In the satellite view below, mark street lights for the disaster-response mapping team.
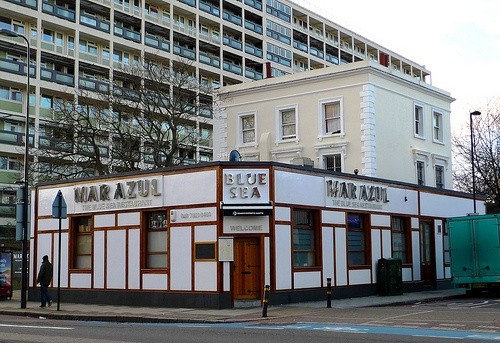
[0,29,32,308]
[470,111,482,214]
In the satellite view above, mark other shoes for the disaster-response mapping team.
[48,300,52,307]
[39,305,45,308]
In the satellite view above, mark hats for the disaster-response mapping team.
[42,255,47,261]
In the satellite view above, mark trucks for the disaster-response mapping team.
[446,213,500,298]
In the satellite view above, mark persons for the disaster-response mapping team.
[36,255,54,308]
